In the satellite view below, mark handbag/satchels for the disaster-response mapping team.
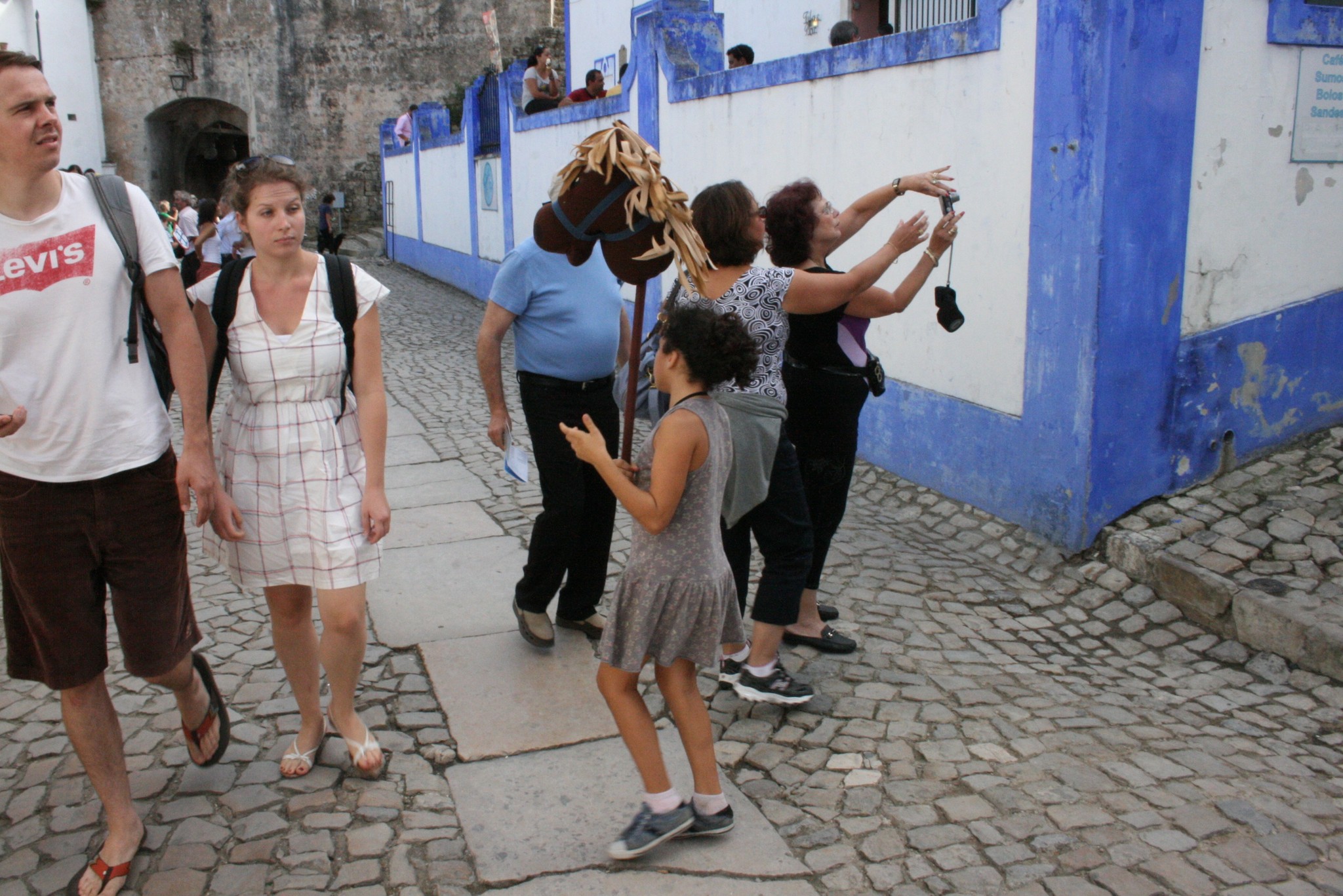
[173,245,184,258]
[863,352,888,397]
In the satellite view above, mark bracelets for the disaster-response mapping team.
[886,242,898,264]
[925,247,939,268]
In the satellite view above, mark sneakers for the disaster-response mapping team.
[608,802,696,861]
[719,640,784,691]
[673,799,737,838]
[513,595,556,647]
[555,610,608,640]
[733,665,814,707]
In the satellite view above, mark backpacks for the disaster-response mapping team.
[86,171,176,414]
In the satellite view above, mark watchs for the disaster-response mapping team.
[892,178,905,196]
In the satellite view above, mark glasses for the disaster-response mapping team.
[856,35,861,40]
[750,207,769,218]
[234,153,296,173]
[819,200,833,216]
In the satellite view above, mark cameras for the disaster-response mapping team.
[940,190,959,218]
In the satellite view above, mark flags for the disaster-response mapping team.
[482,10,503,73]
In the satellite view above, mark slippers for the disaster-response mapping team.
[179,652,231,768]
[67,824,148,896]
[326,701,386,778]
[279,714,328,780]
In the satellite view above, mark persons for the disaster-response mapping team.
[185,154,389,779]
[0,51,232,896]
[521,47,562,115]
[726,44,754,68]
[768,165,966,650]
[561,297,758,860]
[830,21,861,47]
[63,164,337,285]
[669,179,929,705]
[558,70,607,108]
[605,63,629,96]
[394,104,418,147]
[479,233,639,647]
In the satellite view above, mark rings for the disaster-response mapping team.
[948,229,955,236]
[918,228,924,235]
[932,172,938,184]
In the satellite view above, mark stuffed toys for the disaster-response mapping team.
[533,121,719,296]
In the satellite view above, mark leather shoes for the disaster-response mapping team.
[781,624,856,654]
[815,600,838,621]
